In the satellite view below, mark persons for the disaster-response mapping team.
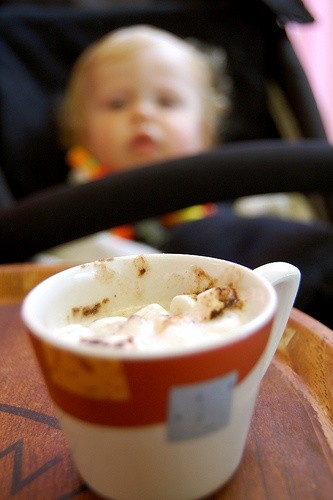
[39,19,316,265]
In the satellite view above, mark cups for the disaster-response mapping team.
[20,252,301,500]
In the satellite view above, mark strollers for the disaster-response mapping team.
[0,0,333,331]
